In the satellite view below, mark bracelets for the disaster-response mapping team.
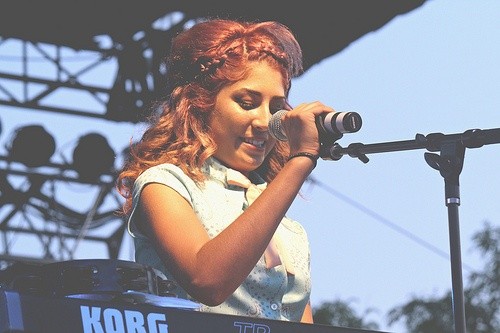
[287,149,318,174]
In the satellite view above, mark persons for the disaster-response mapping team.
[109,16,335,324]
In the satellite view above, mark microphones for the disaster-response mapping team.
[268,109,363,142]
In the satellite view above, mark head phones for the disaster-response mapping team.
[1,261,178,305]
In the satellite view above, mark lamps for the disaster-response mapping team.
[5,123,57,168]
[71,133,116,182]
